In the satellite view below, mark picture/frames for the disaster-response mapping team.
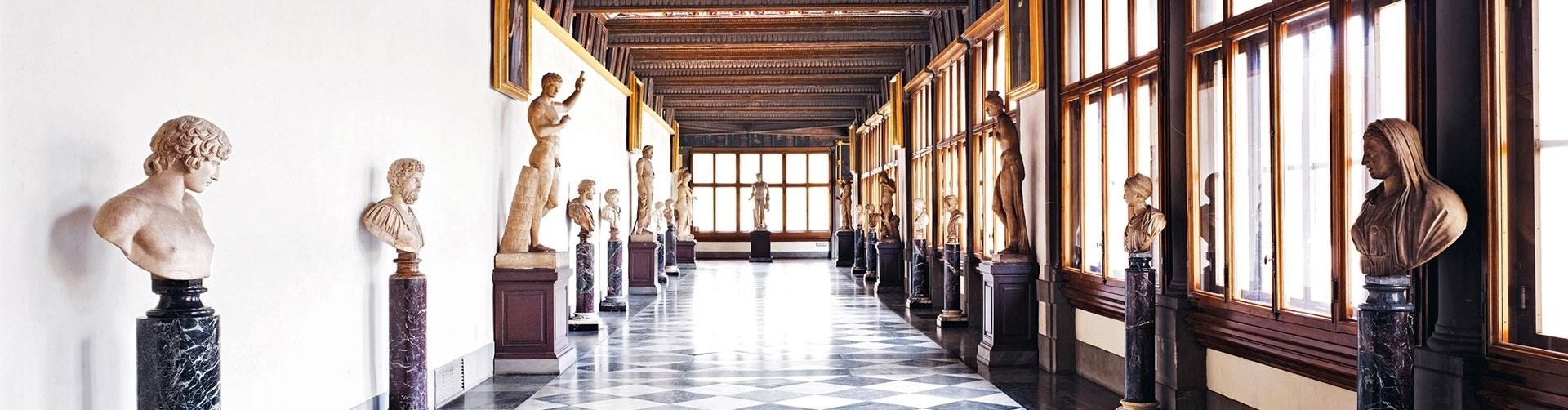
[491,0,533,103]
[1002,0,1044,102]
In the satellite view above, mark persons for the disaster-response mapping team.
[748,173,772,229]
[910,197,930,239]
[1350,118,1470,276]
[358,159,430,254]
[525,70,698,258]
[985,90,1032,255]
[1123,173,1168,254]
[1198,172,1215,252]
[92,115,235,280]
[837,171,897,239]
[944,194,965,243]
